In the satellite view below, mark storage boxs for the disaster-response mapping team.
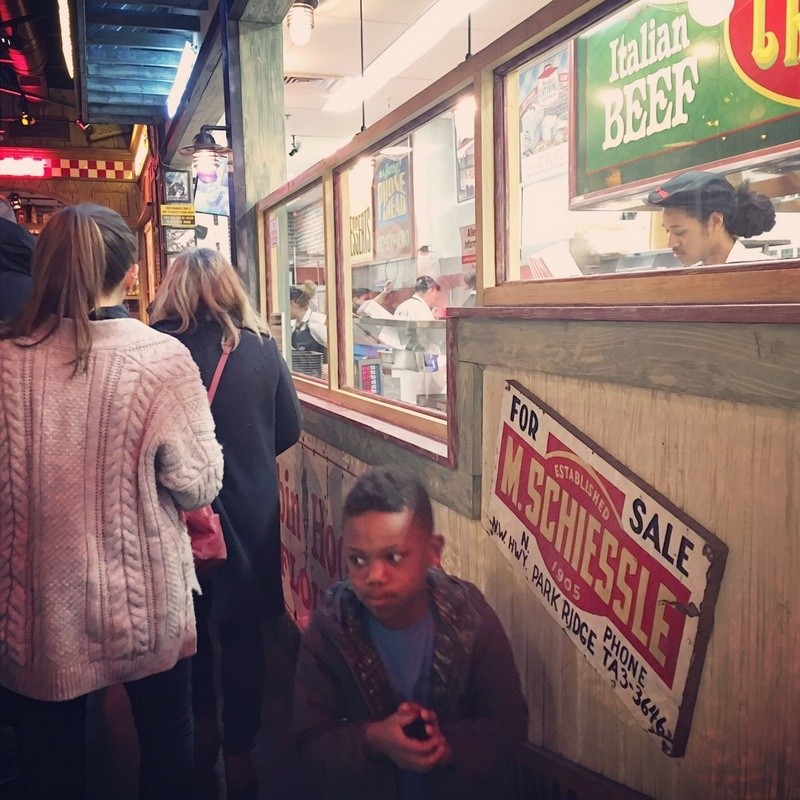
[357,299,411,349]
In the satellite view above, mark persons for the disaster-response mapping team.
[285,267,480,367]
[138,243,307,789]
[640,169,780,272]
[286,464,529,800]
[0,202,224,800]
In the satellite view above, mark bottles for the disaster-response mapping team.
[31,203,37,224]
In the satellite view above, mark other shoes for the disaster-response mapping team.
[192,718,218,776]
[220,749,261,800]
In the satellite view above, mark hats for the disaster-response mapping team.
[647,171,736,218]
[415,276,441,291]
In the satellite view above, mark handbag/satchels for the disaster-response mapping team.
[184,503,227,568]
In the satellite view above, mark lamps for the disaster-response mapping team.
[74,118,91,130]
[176,123,233,185]
[10,192,22,209]
[284,0,319,47]
[19,112,35,127]
[288,134,301,157]
